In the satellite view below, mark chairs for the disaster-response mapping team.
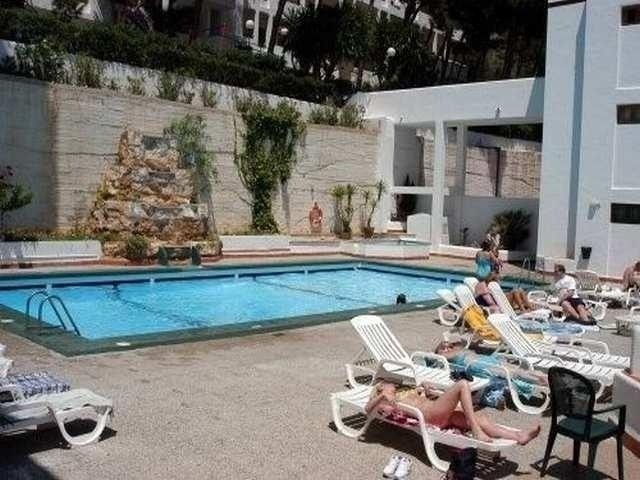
[343,313,492,419]
[437,258,640,415]
[0,370,70,403]
[539,365,629,480]
[0,388,120,448]
[330,385,525,473]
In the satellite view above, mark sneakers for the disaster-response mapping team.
[384,454,413,479]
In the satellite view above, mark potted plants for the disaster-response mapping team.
[334,183,353,239]
[360,179,384,239]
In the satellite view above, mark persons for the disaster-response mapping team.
[475,240,498,287]
[396,294,408,304]
[486,224,501,272]
[554,266,588,321]
[365,379,540,446]
[622,262,640,292]
[475,271,534,313]
[435,342,549,387]
